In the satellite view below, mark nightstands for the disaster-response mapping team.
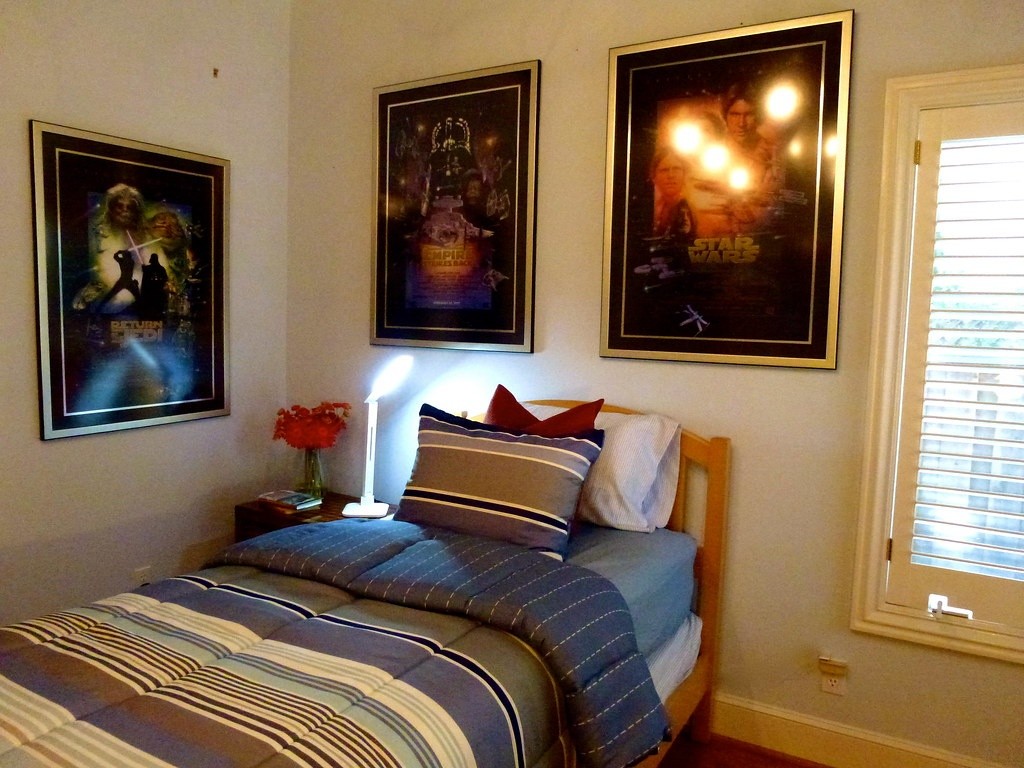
[232,492,366,544]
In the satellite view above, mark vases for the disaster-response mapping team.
[302,448,322,499]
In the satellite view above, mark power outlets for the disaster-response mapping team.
[818,656,849,696]
[135,565,152,588]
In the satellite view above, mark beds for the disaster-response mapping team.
[0,399,731,768]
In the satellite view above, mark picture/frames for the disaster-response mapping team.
[30,121,233,443]
[368,59,543,355]
[600,9,855,371]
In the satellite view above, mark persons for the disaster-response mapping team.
[651,83,792,241]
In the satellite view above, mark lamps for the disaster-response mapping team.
[342,353,411,518]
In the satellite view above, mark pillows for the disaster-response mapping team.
[389,404,605,562]
[522,402,682,533]
[483,385,604,437]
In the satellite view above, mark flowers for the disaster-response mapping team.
[272,400,352,493]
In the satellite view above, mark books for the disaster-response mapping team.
[258,489,324,510]
[269,505,321,515]
